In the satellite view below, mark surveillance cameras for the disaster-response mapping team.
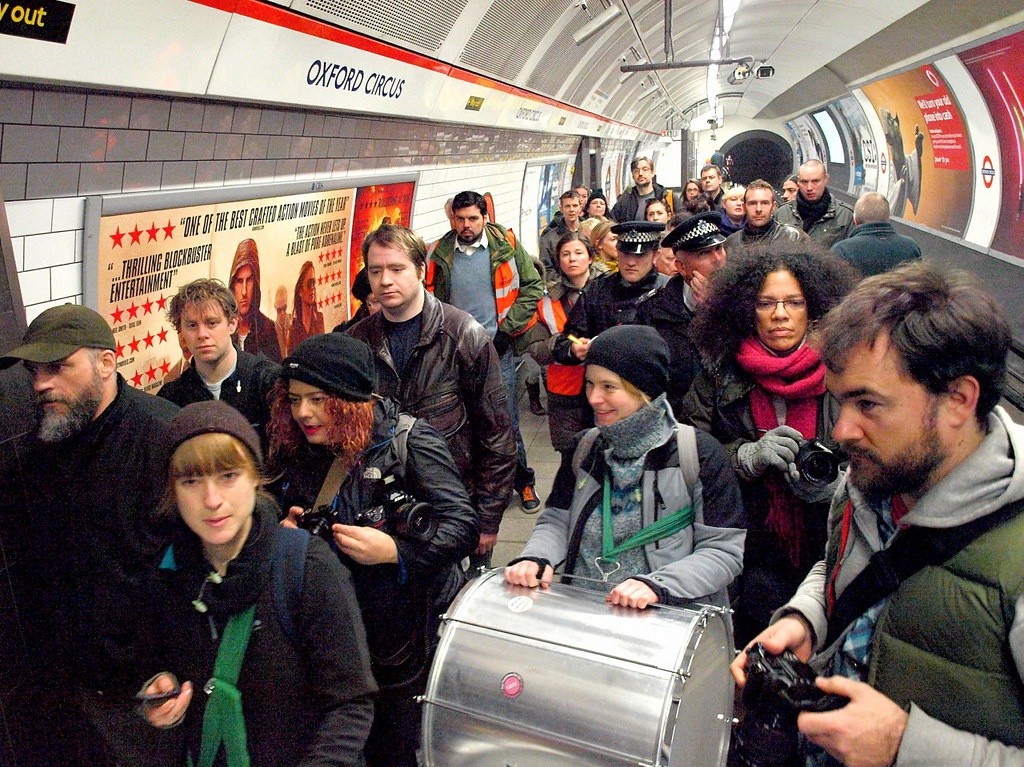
[755,66,775,79]
[727,67,749,85]
[707,117,718,125]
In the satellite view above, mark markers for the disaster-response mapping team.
[567,334,582,345]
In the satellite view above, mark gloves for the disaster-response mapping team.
[736,425,803,478]
[784,438,845,505]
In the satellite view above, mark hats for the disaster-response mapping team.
[160,400,264,487]
[586,323,672,398]
[610,221,666,255]
[586,191,607,207]
[661,210,727,255]
[0,303,116,369]
[351,267,372,302]
[591,221,616,247]
[281,332,377,401]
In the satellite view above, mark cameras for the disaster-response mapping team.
[297,505,347,542]
[727,643,846,767]
[789,436,840,487]
[353,489,442,545]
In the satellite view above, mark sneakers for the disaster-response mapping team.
[518,484,541,514]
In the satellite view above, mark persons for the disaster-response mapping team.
[227,239,282,363]
[164,323,194,383]
[284,260,326,357]
[273,284,293,360]
[1,158,1024,767]
[880,107,924,217]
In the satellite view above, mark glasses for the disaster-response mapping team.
[645,197,668,205]
[301,278,317,289]
[752,298,807,313]
[365,297,383,311]
[275,304,287,313]
[688,188,698,193]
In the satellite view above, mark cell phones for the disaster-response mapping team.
[129,688,184,706]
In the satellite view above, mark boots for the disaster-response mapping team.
[525,377,545,415]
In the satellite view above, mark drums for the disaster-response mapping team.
[415,567,738,767]
